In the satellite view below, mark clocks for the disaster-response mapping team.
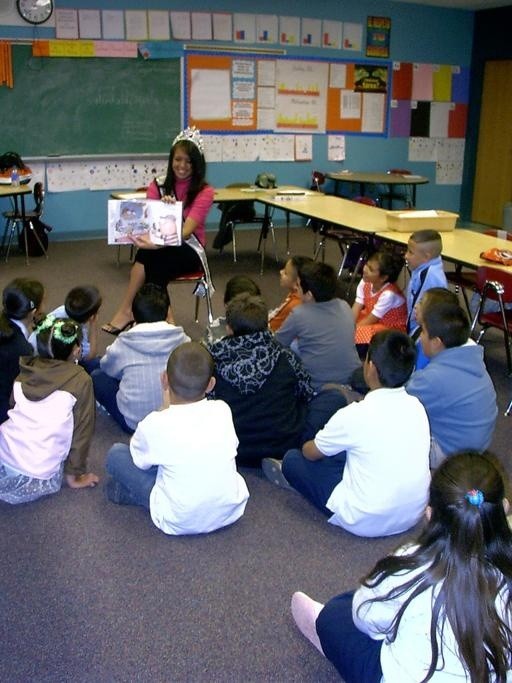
[17,0,54,26]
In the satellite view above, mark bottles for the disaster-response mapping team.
[11,166,21,189]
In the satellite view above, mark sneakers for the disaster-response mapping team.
[103,477,145,507]
[261,457,298,494]
[290,590,326,659]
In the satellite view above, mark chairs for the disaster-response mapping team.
[165,271,215,329]
[1,182,50,266]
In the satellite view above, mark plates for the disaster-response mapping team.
[478,247,512,266]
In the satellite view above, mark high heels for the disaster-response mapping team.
[100,319,136,336]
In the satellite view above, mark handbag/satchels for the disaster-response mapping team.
[0,151,33,188]
[18,203,53,258]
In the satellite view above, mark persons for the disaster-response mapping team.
[100,137,215,336]
[291,451,512,683]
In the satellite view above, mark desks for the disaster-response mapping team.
[374,226,510,376]
[0,184,32,268]
[109,172,428,297]
